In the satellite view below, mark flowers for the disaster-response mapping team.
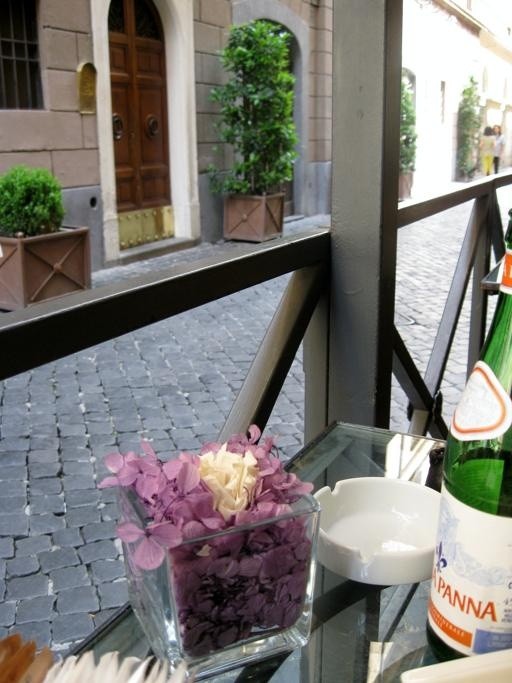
[97,423,316,658]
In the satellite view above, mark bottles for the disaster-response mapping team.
[425,202,512,663]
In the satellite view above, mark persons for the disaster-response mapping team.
[478,126,495,177]
[491,125,506,174]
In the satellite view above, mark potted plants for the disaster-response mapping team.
[0,165,91,311]
[206,19,301,244]
[455,75,482,183]
[398,77,416,204]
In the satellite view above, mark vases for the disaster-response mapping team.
[116,484,322,683]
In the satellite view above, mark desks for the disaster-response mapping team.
[481,254,507,295]
[65,420,448,682]
